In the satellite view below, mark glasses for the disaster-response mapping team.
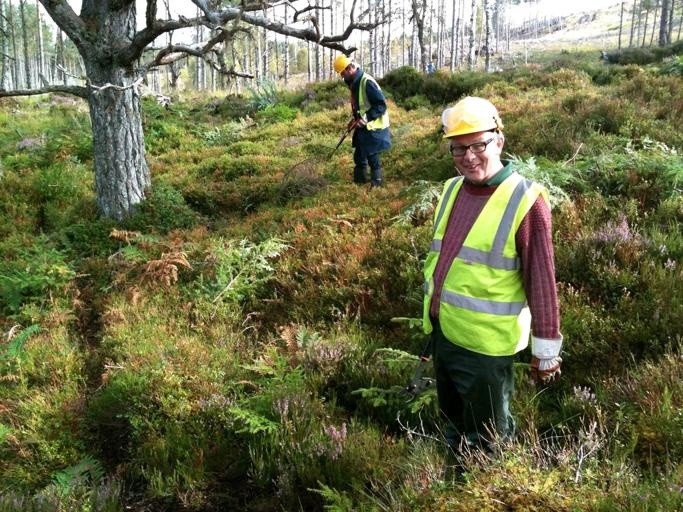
[448,136,497,158]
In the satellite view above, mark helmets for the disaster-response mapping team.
[441,95,505,141]
[333,55,352,74]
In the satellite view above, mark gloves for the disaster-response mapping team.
[525,355,564,390]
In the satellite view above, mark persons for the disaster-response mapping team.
[333,55,394,188]
[417,92,563,495]
